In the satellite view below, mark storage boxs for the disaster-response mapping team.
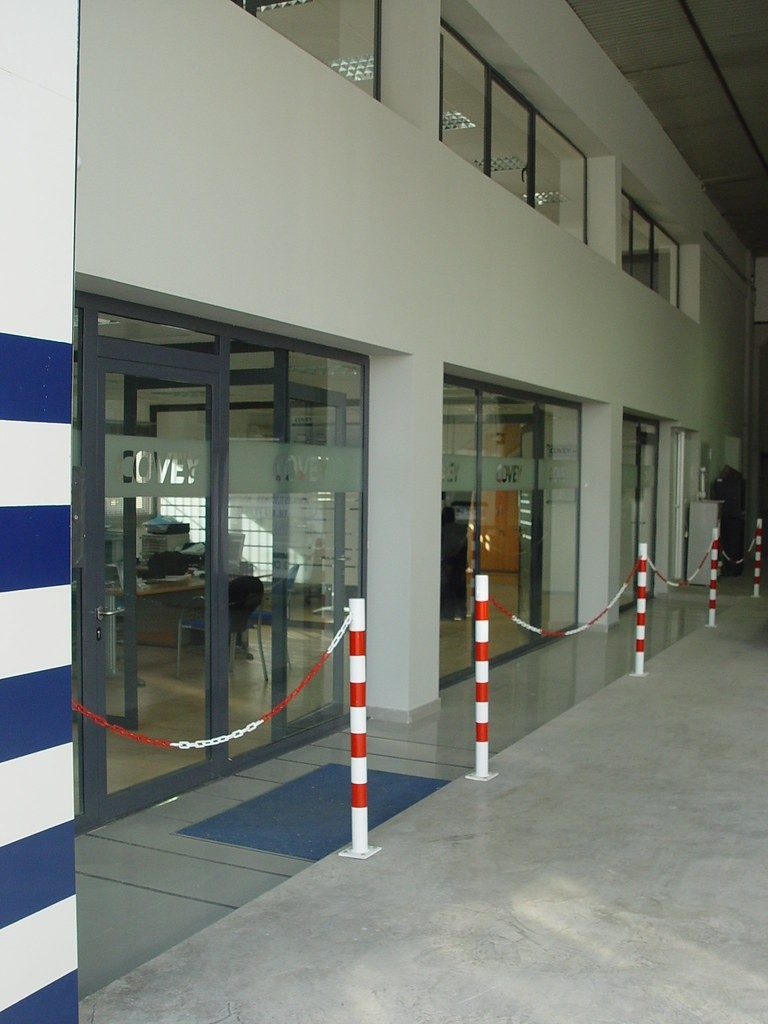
[145,524,189,535]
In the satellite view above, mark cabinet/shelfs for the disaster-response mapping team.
[686,498,724,585]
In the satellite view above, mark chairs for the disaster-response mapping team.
[173,562,301,686]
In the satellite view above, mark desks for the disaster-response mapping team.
[104,571,248,677]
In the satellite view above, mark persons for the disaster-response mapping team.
[440,508,467,621]
